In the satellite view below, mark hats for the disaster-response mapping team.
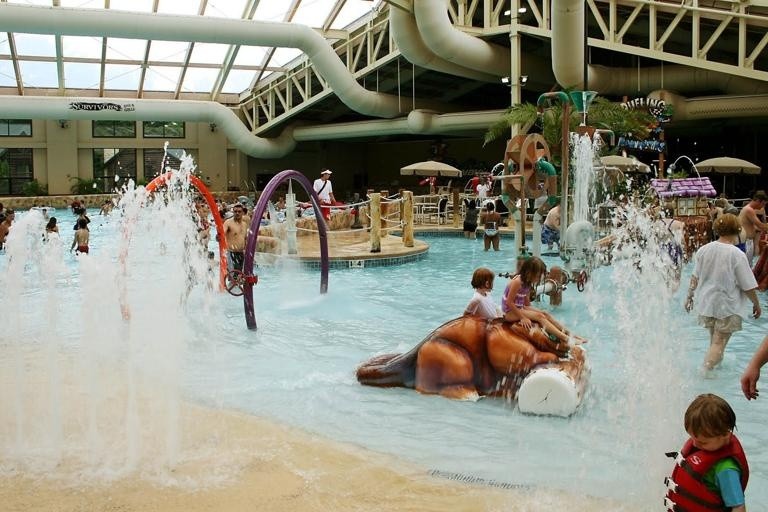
[320,170,332,174]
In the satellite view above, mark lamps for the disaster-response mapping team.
[499,72,528,89]
[503,5,528,18]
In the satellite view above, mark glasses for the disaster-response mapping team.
[234,211,241,213]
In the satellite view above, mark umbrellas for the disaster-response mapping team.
[689,157,759,198]
[595,154,652,177]
[399,161,460,194]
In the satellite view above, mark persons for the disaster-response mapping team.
[191,195,286,280]
[740,334,767,401]
[653,189,767,268]
[312,168,337,223]
[42,195,112,255]
[662,393,748,512]
[501,256,587,345]
[0,204,15,249]
[686,213,760,370]
[463,176,562,251]
[463,268,501,319]
[334,193,366,217]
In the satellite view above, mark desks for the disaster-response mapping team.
[413,202,438,224]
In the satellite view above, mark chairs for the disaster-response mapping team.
[524,196,547,222]
[411,205,418,225]
[420,198,449,226]
[494,198,508,227]
